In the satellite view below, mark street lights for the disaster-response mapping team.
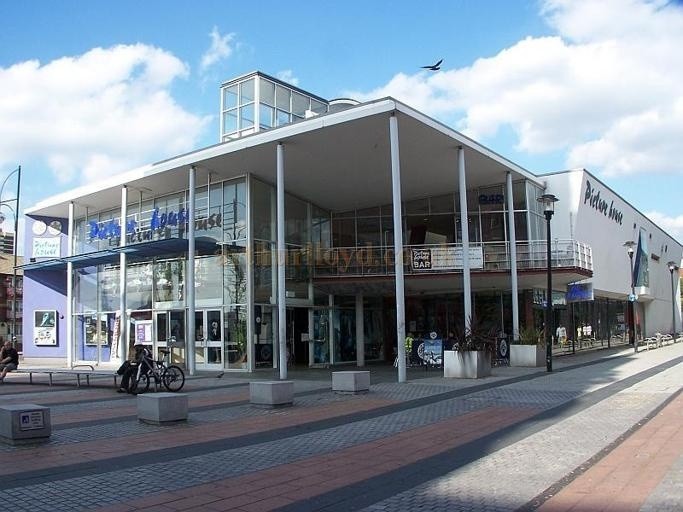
[0,164,21,349]
[536,194,560,372]
[622,240,638,352]
[666,261,676,343]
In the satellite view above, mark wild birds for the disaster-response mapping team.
[419,60,442,70]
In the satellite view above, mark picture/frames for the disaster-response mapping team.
[34,310,58,346]
[83,310,109,346]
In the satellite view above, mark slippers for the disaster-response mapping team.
[116,388,126,393]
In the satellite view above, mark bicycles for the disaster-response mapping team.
[126,350,185,395]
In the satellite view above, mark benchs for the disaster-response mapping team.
[8,364,121,388]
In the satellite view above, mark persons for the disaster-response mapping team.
[112,342,153,393]
[0,340,19,383]
[556,322,567,348]
[405,331,459,371]
[577,322,592,339]
